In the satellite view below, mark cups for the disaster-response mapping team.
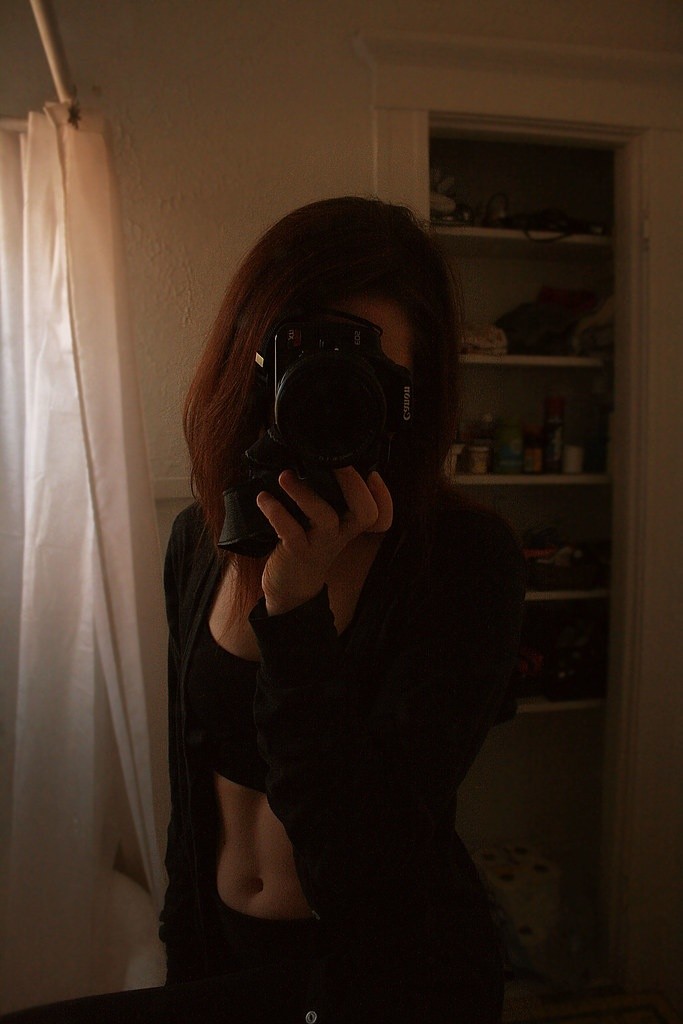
[469,446,490,474]
[565,446,582,473]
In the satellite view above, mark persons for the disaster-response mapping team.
[157,196,526,1024]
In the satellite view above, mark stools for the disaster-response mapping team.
[473,843,564,974]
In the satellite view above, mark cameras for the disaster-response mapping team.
[271,323,414,525]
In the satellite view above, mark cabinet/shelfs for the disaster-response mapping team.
[425,110,626,721]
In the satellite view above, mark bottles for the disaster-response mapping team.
[544,396,564,474]
